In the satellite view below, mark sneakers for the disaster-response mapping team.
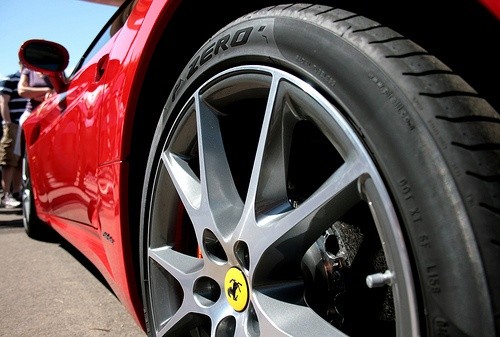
[1,193,21,209]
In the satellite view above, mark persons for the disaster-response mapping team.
[17,66,70,202]
[0,57,43,209]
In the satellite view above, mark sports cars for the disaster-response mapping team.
[18,0,500,337]
